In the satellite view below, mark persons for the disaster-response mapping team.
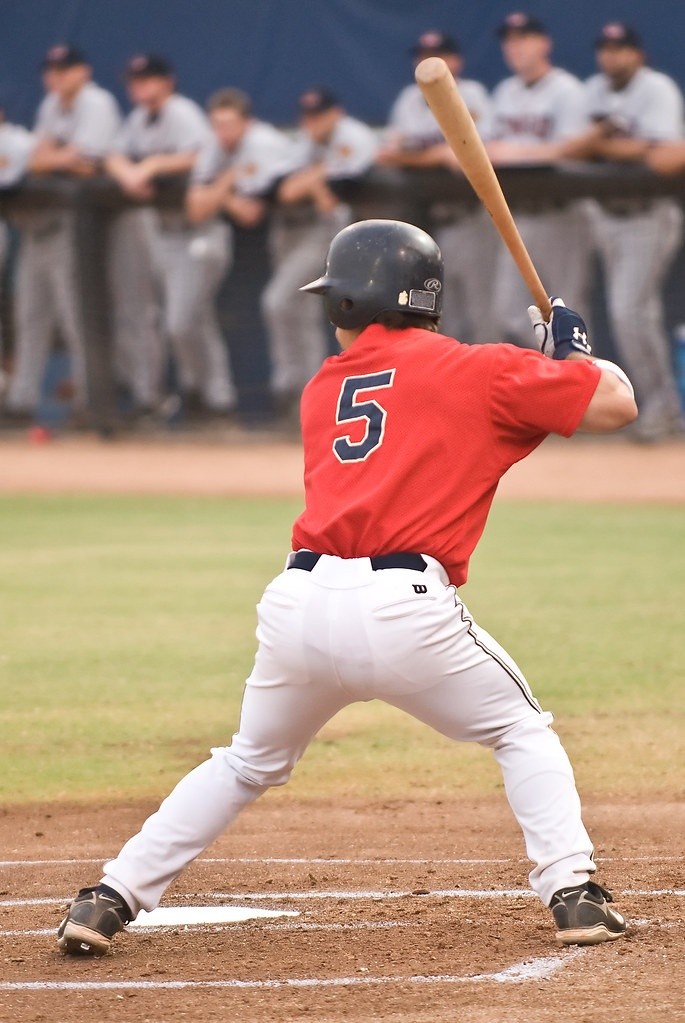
[57,218,642,955]
[0,14,685,444]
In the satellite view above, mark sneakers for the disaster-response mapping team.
[548,880,626,942]
[56,886,123,956]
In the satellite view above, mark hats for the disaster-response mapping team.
[296,89,338,114]
[37,46,84,69]
[496,10,544,36]
[591,23,642,47]
[406,29,459,54]
[122,55,169,75]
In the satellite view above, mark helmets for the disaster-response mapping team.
[297,218,445,330]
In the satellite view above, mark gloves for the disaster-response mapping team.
[526,296,592,360]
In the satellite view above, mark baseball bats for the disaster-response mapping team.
[414,56,554,323]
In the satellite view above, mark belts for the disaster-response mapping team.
[286,550,428,572]
